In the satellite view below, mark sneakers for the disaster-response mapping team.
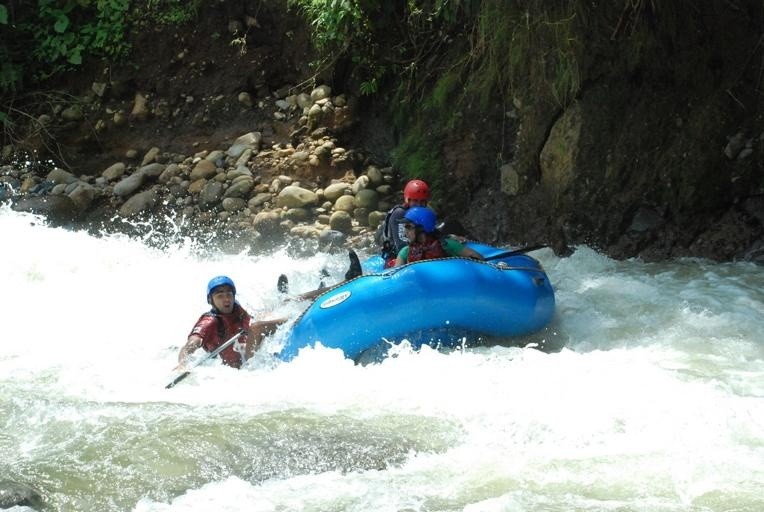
[345,250,362,280]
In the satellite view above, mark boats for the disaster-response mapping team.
[273,239,557,369]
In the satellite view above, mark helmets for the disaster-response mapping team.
[403,206,435,233]
[404,180,432,199]
[207,275,236,304]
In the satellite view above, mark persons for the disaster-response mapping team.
[383,179,484,267]
[172,275,288,372]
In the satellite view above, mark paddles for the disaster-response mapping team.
[483,244,548,260]
[162,328,248,390]
